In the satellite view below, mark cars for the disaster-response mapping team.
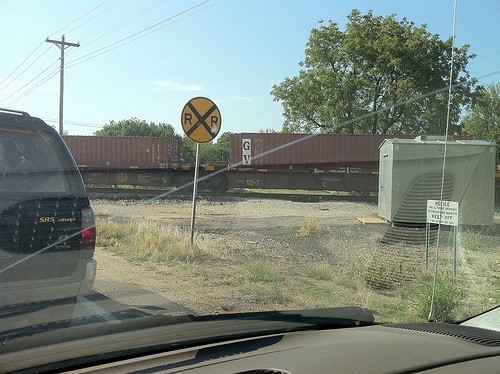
[0,106,99,338]
